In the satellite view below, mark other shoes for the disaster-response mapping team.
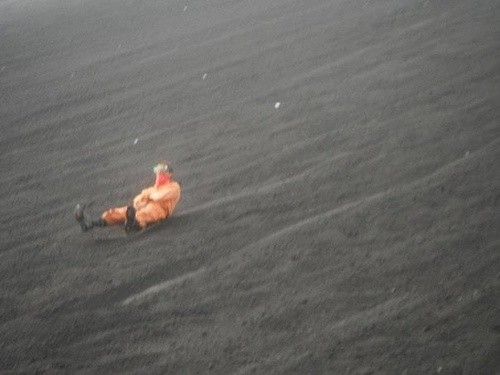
[74,204,94,232]
[124,207,136,234]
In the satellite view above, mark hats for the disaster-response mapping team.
[154,164,173,173]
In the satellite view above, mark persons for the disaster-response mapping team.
[74,162,182,236]
